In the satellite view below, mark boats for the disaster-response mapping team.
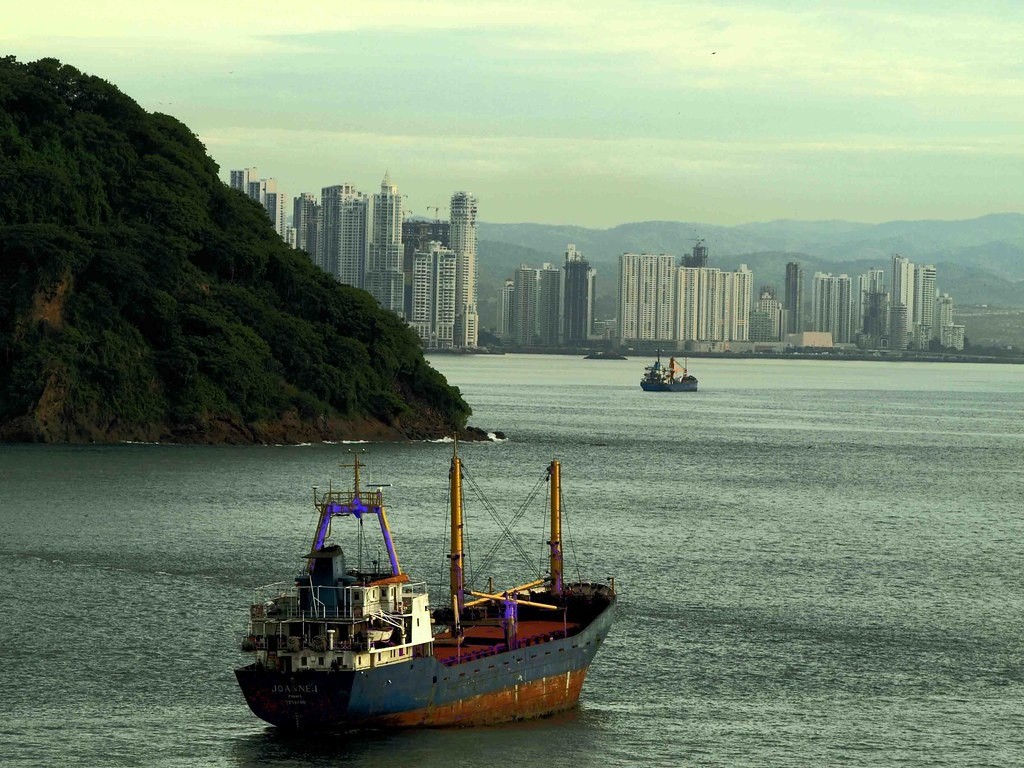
[640,348,700,393]
[232,430,619,736]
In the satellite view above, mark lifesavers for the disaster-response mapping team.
[354,605,362,617]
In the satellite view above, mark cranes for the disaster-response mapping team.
[686,234,705,246]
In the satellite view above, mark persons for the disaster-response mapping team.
[375,488,382,506]
